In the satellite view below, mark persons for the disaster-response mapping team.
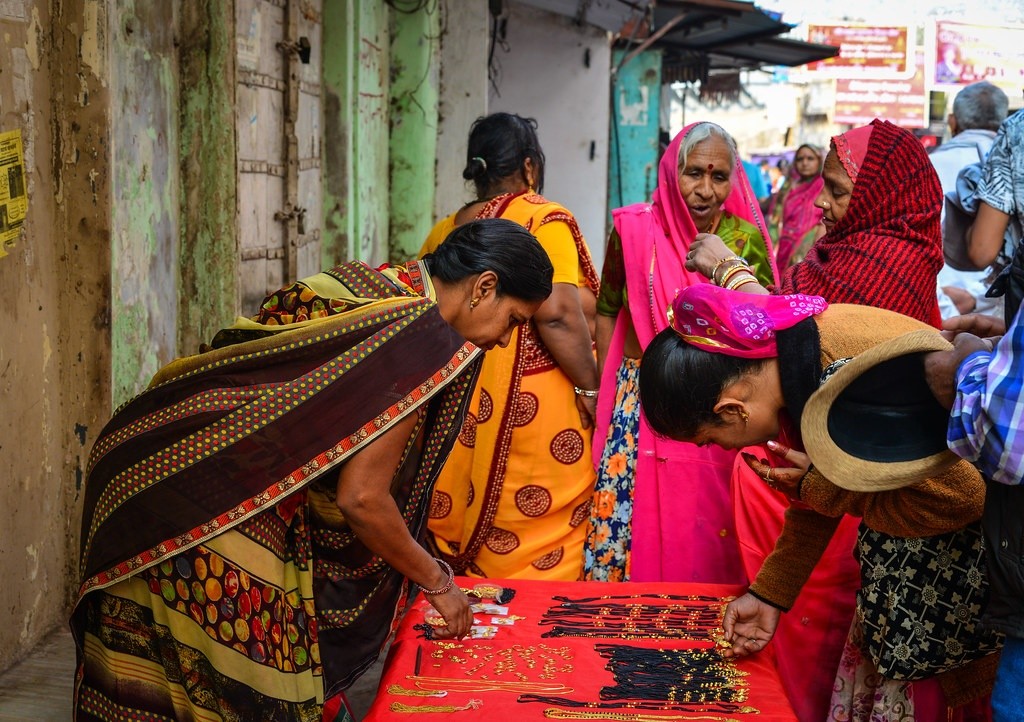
[640,80,1024,722]
[415,113,601,580]
[62,217,554,722]
[581,122,782,585]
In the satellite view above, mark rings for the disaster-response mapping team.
[687,251,692,260]
[763,468,774,482]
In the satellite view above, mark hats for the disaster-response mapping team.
[801,330,963,492]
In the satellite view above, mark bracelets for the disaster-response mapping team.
[713,255,748,281]
[726,275,756,289]
[719,265,746,286]
[732,279,759,290]
[574,386,597,397]
[414,557,454,594]
[721,267,754,288]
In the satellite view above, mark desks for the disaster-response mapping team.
[364,575,799,722]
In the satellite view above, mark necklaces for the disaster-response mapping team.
[385,594,760,722]
